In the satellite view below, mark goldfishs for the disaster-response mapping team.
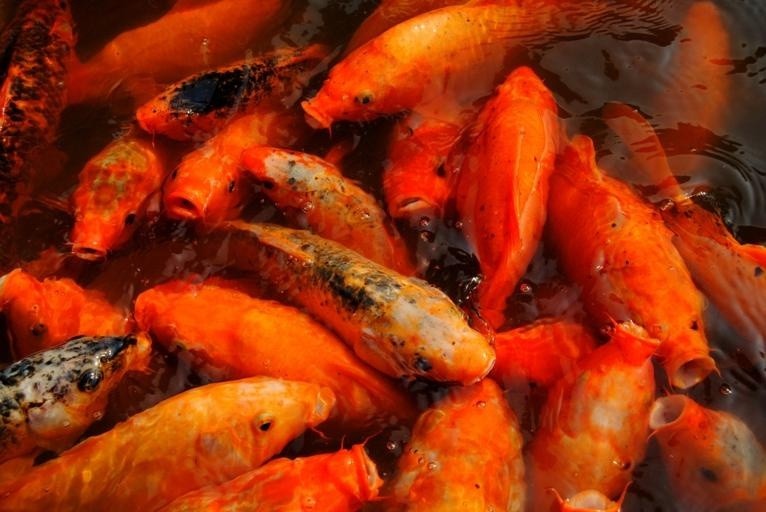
[1,2,766,511]
